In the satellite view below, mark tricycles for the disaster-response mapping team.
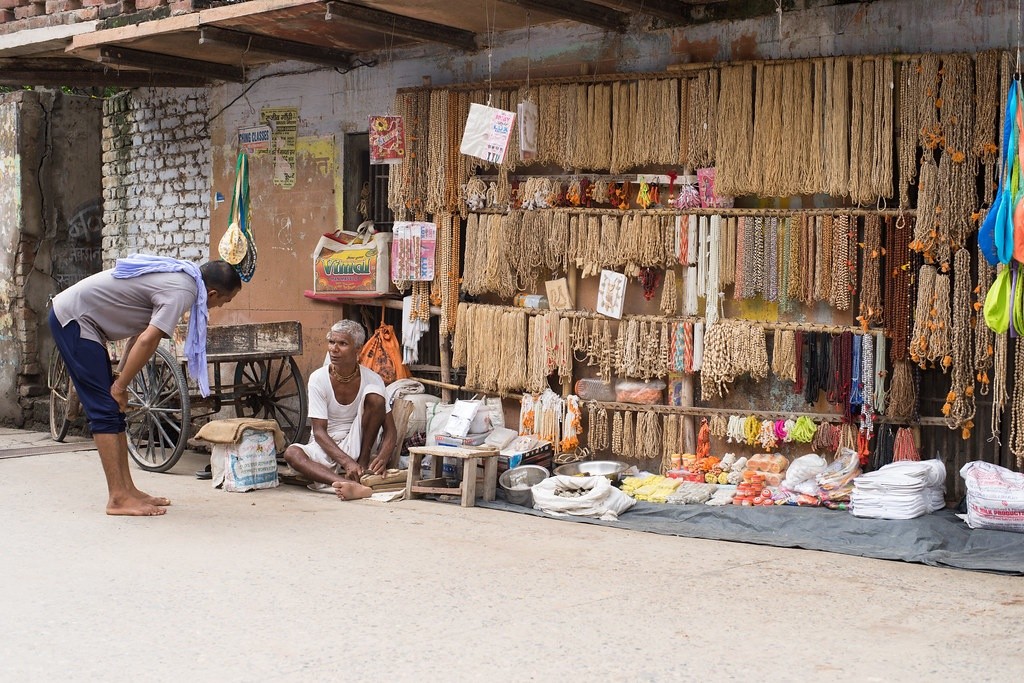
[46,295,308,474]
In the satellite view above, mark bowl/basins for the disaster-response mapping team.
[499,464,550,507]
[553,460,629,490]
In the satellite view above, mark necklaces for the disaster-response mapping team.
[708,51,1024,470]
[332,365,358,383]
[385,82,463,341]
[452,214,709,477]
[463,75,712,175]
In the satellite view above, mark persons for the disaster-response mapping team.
[283,320,397,501]
[48,261,242,516]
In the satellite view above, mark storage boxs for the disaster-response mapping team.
[433,428,493,479]
[471,438,552,492]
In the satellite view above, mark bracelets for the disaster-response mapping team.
[696,411,870,465]
[462,175,712,211]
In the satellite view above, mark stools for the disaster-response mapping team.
[407,446,499,507]
[195,418,287,491]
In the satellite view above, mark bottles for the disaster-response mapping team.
[574,377,615,402]
[513,292,548,308]
